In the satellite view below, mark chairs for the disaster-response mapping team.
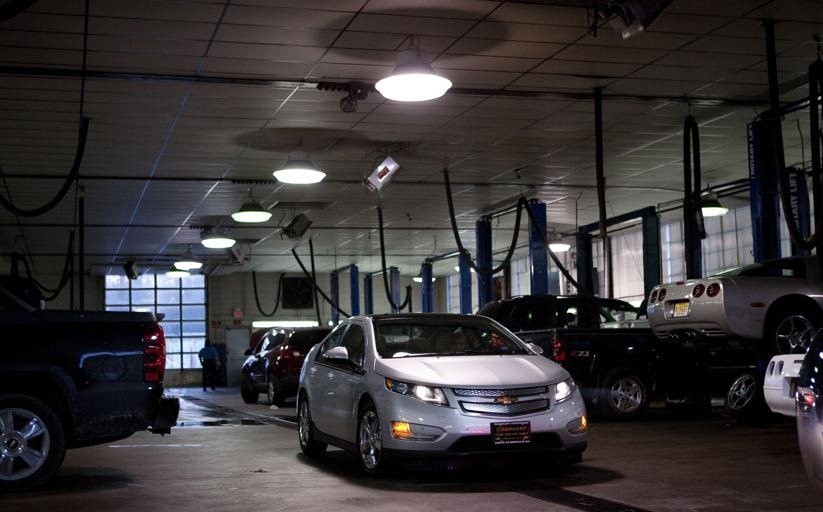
[344,335,468,362]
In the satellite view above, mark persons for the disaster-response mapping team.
[447,333,469,353]
[198,339,220,392]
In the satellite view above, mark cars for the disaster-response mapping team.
[646,258,822,366]
[0,281,181,494]
[294,311,591,479]
[764,348,806,417]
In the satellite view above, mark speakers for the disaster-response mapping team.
[286,213,312,237]
[366,156,401,193]
[123,262,138,279]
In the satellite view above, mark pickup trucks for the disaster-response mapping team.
[513,300,764,419]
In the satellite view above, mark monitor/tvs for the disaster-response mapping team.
[282,277,314,309]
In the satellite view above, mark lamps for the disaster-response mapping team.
[272,138,327,185]
[701,188,729,219]
[375,34,453,102]
[200,225,237,249]
[231,183,273,223]
[166,259,190,277]
[174,244,204,270]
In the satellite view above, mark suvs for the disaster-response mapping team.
[474,293,641,333]
[239,326,336,406]
